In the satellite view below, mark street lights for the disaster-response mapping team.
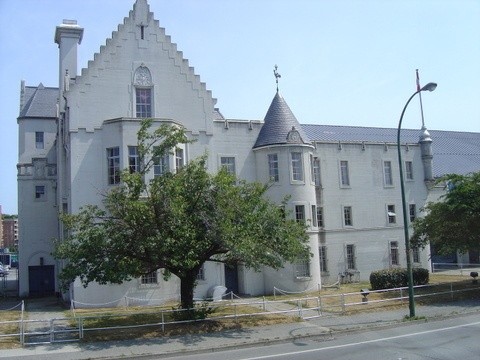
[396,82,438,317]
